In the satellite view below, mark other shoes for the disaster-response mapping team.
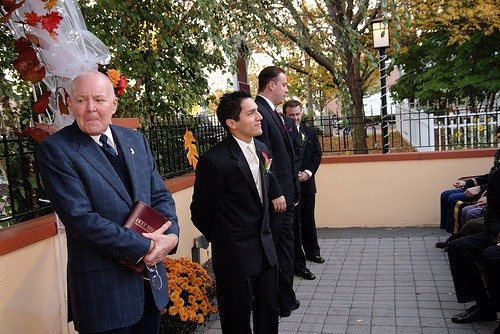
[435,242,448,248]
[280,300,300,317]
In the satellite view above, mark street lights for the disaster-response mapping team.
[371,17,393,154]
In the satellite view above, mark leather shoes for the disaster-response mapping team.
[451,305,497,323]
[302,268,316,280]
[306,256,325,263]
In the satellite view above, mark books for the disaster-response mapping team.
[113,201,172,272]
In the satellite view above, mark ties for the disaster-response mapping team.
[248,144,263,204]
[99,134,117,156]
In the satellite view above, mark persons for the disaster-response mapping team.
[274,104,316,280]
[35,70,179,334]
[253,66,300,317]
[189,90,279,334]
[435,125,500,334]
[282,99,325,263]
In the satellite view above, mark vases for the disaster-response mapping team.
[165,300,199,334]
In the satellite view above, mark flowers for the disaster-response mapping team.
[260,149,272,175]
[159,255,218,325]
[299,132,306,143]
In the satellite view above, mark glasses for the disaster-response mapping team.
[136,255,162,290]
